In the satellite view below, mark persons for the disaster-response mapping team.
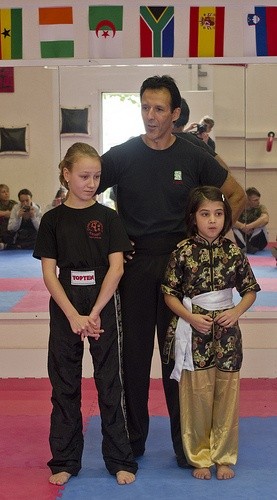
[182,116,214,151]
[230,187,269,255]
[91,74,247,469]
[52,187,68,208]
[168,97,230,174]
[160,185,261,481]
[7,188,43,251]
[31,142,139,487]
[0,184,20,251]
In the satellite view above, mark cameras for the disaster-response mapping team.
[197,124,207,134]
[23,205,30,213]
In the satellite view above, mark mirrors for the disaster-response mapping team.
[0,57,277,320]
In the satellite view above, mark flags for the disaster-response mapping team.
[0,5,277,60]
